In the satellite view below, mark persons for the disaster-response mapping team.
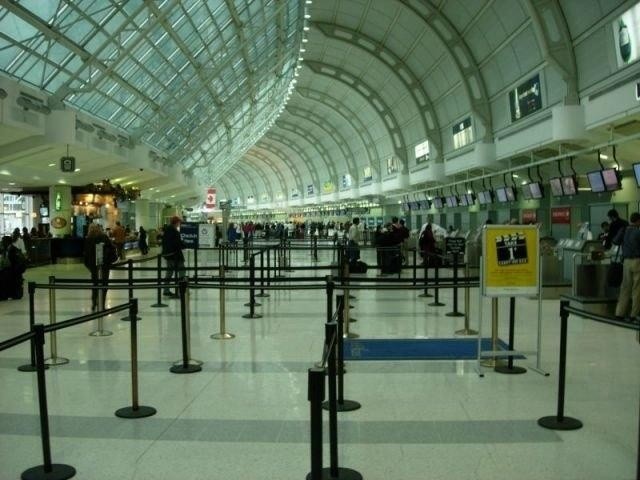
[479,219,496,224]
[600,209,629,263]
[509,217,521,224]
[596,221,610,252]
[163,218,186,297]
[228,218,284,246]
[1,227,36,300]
[83,221,116,310]
[349,216,456,273]
[111,220,147,259]
[612,211,640,326]
[284,218,352,241]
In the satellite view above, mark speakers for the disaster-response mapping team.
[60,156,75,172]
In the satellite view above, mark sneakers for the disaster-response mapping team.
[92,303,106,311]
[163,291,175,296]
[615,314,639,325]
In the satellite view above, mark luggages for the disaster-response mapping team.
[428,248,442,268]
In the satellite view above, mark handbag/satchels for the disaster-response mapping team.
[606,262,623,289]
[349,261,367,273]
